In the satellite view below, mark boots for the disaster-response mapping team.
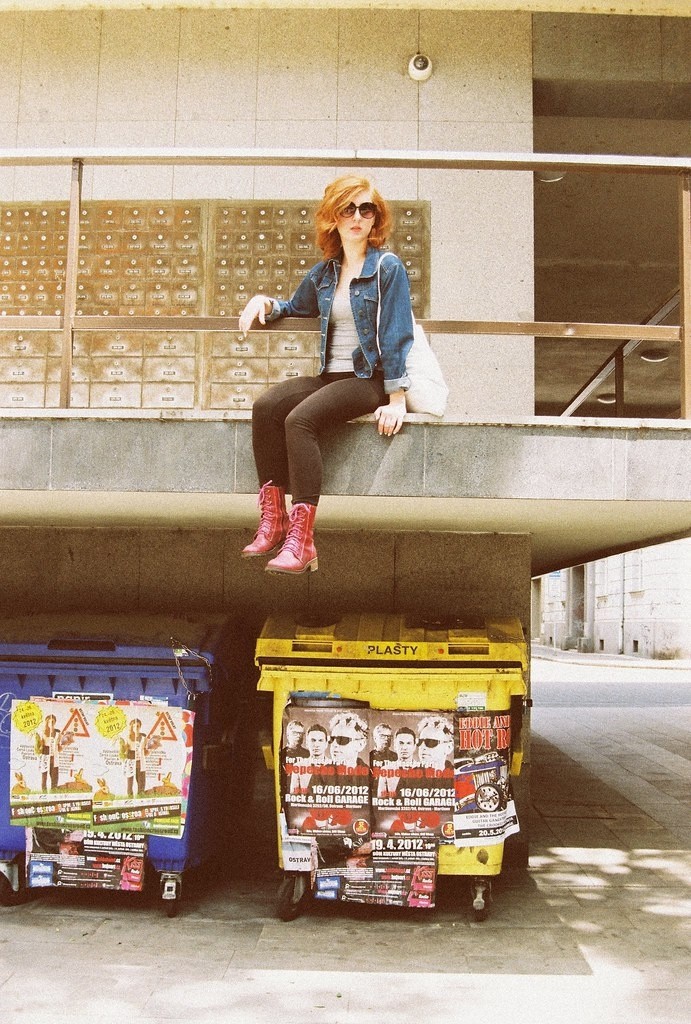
[241,480,289,558]
[265,503,319,574]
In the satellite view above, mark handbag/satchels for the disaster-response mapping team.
[375,253,450,417]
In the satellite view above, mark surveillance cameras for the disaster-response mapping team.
[409,53,432,81]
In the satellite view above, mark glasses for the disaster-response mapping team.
[292,731,305,736]
[415,739,451,747]
[340,202,378,219]
[379,734,393,739]
[328,735,363,745]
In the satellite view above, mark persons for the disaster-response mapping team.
[279,712,454,832]
[119,719,148,800]
[240,174,414,576]
[35,715,65,794]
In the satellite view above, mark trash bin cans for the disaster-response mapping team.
[253,607,532,917]
[0,608,236,917]
